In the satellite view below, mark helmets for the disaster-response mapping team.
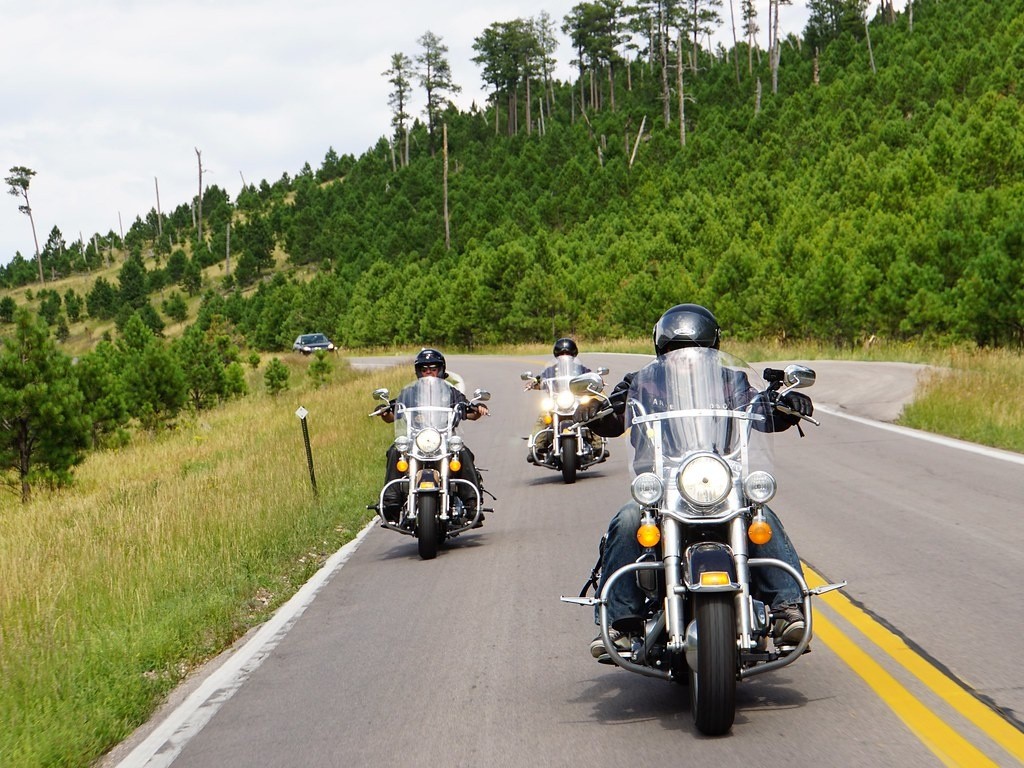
[414,350,446,379]
[653,304,720,359]
[553,338,578,358]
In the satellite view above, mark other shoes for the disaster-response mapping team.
[381,511,396,526]
[527,453,544,463]
[593,448,610,458]
[465,507,485,522]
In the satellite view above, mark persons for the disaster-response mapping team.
[527,338,610,463]
[573,303,814,658]
[374,349,489,522]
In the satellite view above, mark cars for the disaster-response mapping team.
[292,333,335,355]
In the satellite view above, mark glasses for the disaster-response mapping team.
[558,351,573,355]
[420,365,440,372]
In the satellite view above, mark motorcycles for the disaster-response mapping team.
[520,355,612,485]
[368,387,496,564]
[567,366,848,736]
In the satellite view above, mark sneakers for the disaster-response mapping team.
[772,602,812,647]
[590,624,632,658]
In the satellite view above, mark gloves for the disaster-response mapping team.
[781,391,813,426]
[572,398,607,430]
